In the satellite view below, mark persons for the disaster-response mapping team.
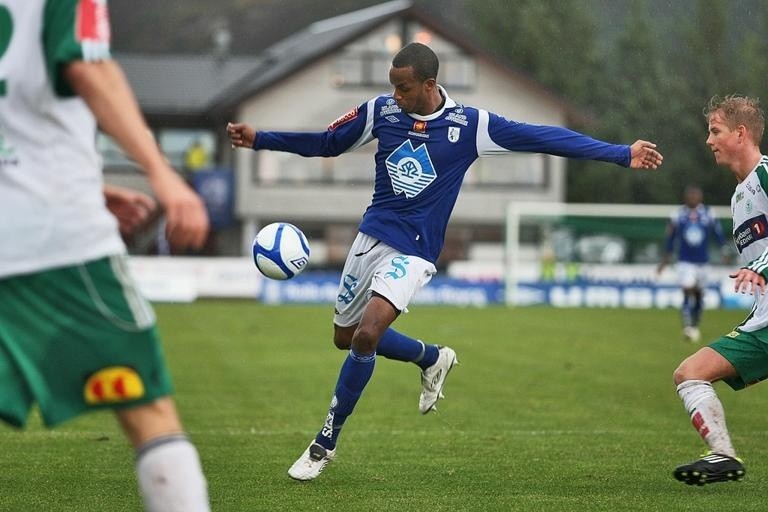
[672,93,768,486]
[655,180,736,348]
[223,41,665,483]
[0,0,213,512]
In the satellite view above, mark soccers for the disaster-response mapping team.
[252,222,310,280]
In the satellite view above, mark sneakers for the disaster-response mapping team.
[287,439,337,481]
[673,450,746,487]
[418,342,461,414]
